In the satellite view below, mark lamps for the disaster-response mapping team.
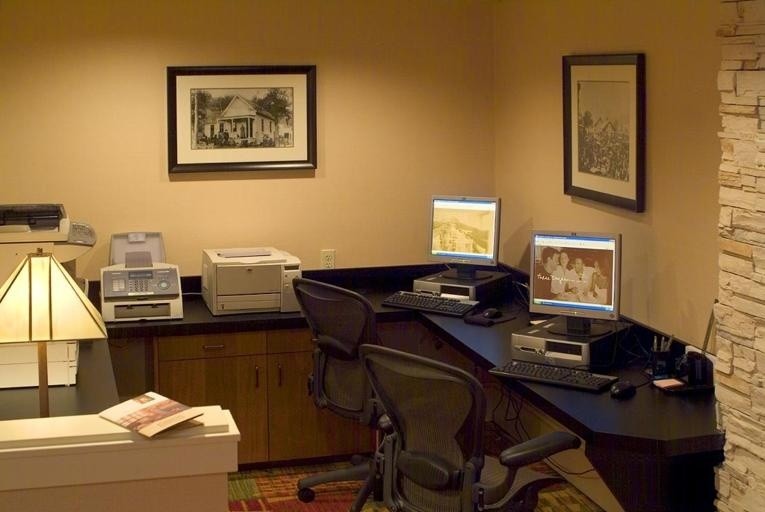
[0,253,107,417]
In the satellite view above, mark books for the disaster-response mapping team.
[217,246,272,258]
[97,390,204,442]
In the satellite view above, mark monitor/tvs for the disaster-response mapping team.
[528,231,623,338]
[429,194,502,280]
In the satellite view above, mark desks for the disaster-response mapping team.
[367,285,724,512]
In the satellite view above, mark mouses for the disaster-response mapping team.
[610,379,636,399]
[483,308,502,318]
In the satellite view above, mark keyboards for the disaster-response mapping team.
[381,291,480,318]
[488,360,618,396]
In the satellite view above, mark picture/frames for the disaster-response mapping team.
[166,64,317,179]
[562,51,646,214]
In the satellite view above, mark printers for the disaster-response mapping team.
[200,247,303,315]
[98,229,185,320]
[1,204,98,302]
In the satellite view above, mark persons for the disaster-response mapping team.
[550,250,581,294]
[534,247,561,299]
[223,129,229,144]
[240,124,246,138]
[590,256,610,303]
[571,257,596,292]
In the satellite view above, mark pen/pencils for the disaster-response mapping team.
[647,335,674,376]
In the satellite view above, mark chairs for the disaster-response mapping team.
[291,278,395,512]
[358,343,581,511]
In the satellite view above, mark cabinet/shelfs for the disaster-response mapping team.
[160,324,376,466]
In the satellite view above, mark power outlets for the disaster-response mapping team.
[319,250,335,270]
